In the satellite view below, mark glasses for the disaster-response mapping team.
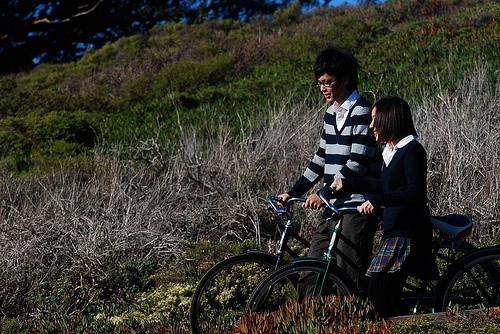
[316,80,341,87]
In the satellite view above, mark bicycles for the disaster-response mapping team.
[188,194,500,334]
[243,185,500,313]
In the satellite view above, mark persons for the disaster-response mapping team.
[330,97,440,318]
[276,46,382,304]
